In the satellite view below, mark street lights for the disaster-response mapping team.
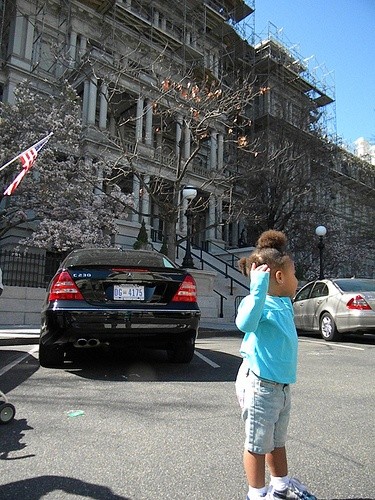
[181,185,197,268]
[314,225,328,279]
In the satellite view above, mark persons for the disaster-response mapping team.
[235,230,317,500]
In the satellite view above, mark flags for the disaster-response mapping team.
[3,137,51,196]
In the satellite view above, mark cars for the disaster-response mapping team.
[293,277,375,341]
[39,247,202,367]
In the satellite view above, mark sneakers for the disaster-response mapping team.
[268,472,317,500]
[247,491,284,500]
